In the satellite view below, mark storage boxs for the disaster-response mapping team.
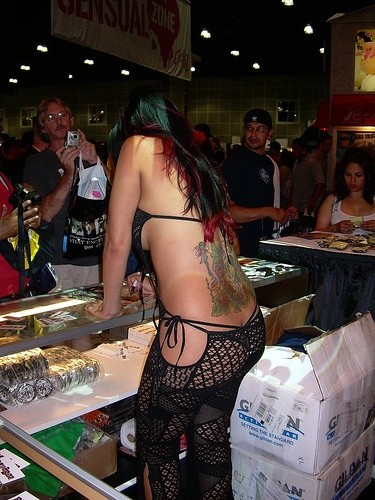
[231,296,375,500]
[44,418,118,497]
[128,320,160,347]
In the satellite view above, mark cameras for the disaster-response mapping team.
[9,184,42,212]
[64,131,79,148]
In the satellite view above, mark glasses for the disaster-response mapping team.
[44,111,69,122]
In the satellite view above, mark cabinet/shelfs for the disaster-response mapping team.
[0,256,307,445]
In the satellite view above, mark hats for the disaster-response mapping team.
[244,108,273,130]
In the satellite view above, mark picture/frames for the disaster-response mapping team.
[88,103,107,126]
[20,107,37,128]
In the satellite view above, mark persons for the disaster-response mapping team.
[0,99,327,302]
[85,97,265,500]
[315,155,375,233]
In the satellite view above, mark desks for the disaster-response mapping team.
[258,230,375,332]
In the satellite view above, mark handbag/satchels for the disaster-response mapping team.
[61,150,116,259]
